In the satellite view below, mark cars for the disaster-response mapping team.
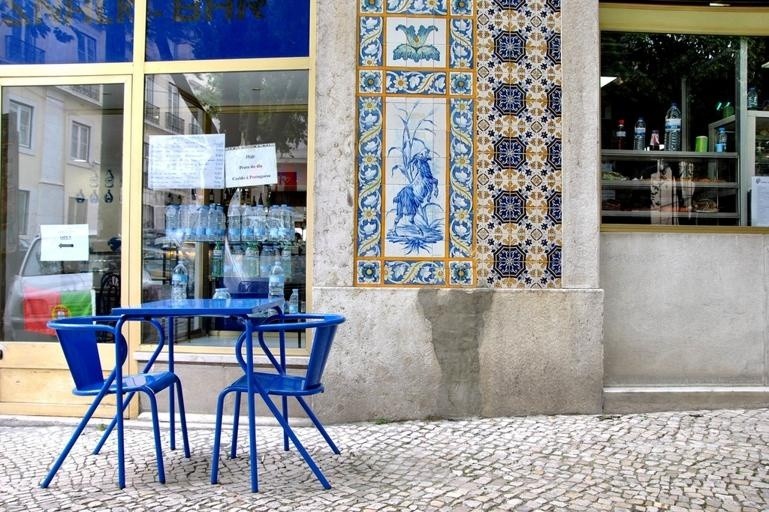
[223,206,306,300]
[3,233,152,341]
[141,231,233,281]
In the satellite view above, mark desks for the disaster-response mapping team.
[110,298,285,493]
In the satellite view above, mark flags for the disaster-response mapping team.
[23,288,94,336]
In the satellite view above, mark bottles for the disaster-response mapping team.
[209,242,304,278]
[165,204,295,241]
[171,260,188,306]
[717,127,727,152]
[617,102,681,152]
[268,262,285,299]
[289,288,300,313]
[747,88,758,109]
[211,288,231,300]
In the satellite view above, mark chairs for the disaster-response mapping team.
[38,314,190,488]
[212,314,345,489]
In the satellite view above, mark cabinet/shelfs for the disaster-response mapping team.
[62,236,306,295]
[708,110,768,227]
[601,149,740,225]
